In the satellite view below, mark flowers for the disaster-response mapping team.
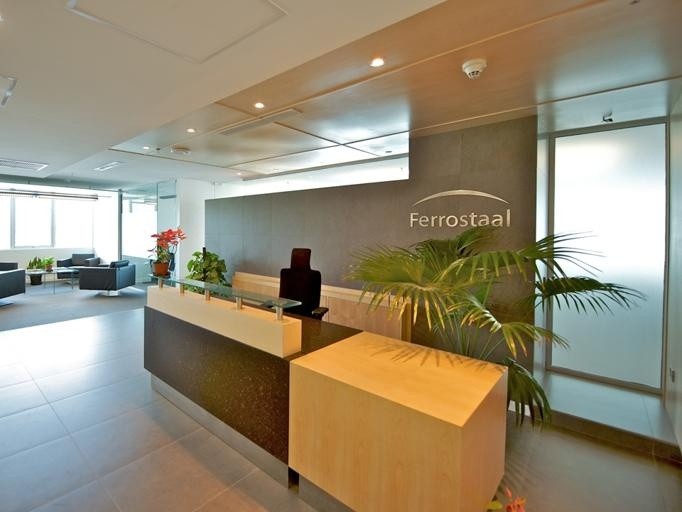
[147,225,187,263]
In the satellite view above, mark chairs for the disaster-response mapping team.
[259,248,329,320]
[57,254,100,285]
[79,260,136,296]
[0,263,26,307]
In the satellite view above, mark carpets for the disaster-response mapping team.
[0,279,147,332]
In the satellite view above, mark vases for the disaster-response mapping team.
[151,261,170,276]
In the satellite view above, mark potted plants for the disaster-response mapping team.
[45,257,54,272]
[27,257,45,285]
